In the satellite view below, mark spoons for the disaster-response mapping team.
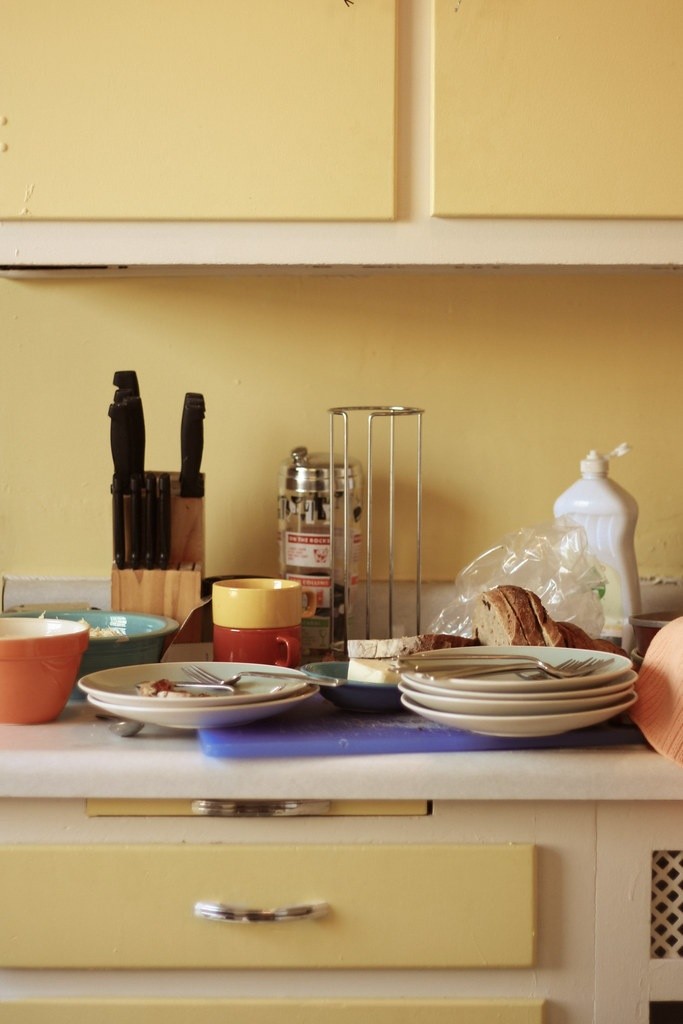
[96,713,143,738]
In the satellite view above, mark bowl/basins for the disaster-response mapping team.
[302,662,405,714]
[1,611,178,698]
[0,616,88,723]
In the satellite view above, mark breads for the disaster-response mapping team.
[472,584,629,663]
[346,633,479,658]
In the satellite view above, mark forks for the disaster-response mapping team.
[387,653,614,680]
[182,664,346,689]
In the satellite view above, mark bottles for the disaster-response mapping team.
[274,448,363,652]
[553,449,643,638]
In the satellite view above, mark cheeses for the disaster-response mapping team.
[347,658,401,684]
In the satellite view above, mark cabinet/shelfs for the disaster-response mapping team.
[0,797,683,1024]
[0,0,683,270]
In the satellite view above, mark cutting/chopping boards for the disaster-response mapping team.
[197,695,640,757]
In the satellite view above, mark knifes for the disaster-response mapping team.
[107,369,206,570]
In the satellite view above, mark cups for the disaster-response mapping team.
[213,576,302,668]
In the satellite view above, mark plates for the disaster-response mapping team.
[76,662,320,730]
[397,645,639,739]
